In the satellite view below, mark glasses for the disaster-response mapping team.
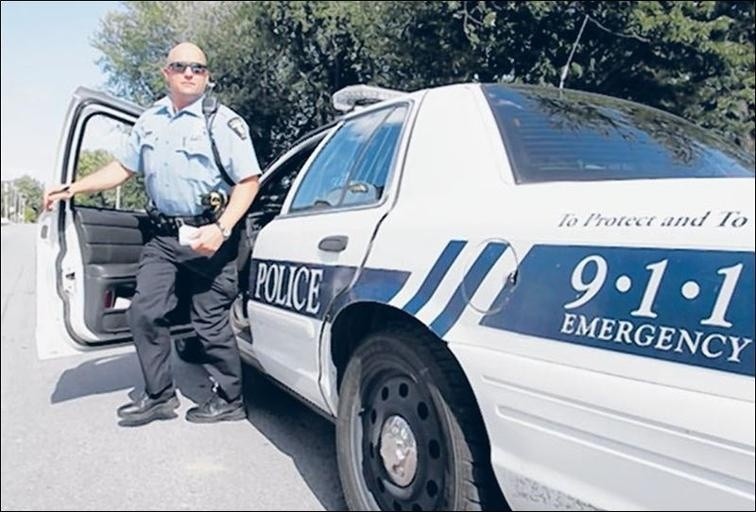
[168,61,207,73]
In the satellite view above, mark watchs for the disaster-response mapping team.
[215,220,232,241]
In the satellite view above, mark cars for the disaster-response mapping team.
[36,78,756,512]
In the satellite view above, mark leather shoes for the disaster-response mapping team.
[118,388,179,421]
[186,395,248,423]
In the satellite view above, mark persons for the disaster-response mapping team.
[308,178,384,210]
[41,41,264,428]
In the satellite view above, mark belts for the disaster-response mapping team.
[168,216,213,236]
